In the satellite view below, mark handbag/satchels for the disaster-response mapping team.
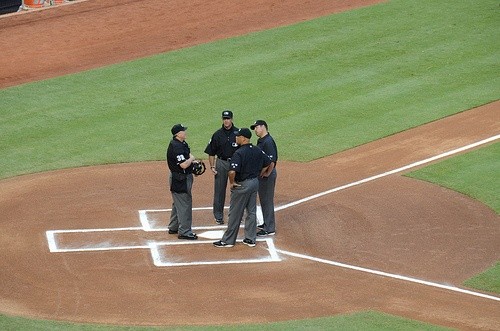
[170,172,188,194]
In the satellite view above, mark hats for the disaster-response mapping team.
[222,111,233,118]
[234,128,252,139]
[171,125,187,135]
[250,120,267,130]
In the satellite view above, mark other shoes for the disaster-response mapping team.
[242,239,256,248]
[213,241,235,248]
[257,224,276,237]
[169,230,198,240]
[215,218,224,225]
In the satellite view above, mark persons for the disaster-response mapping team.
[167,124,206,239]
[250,120,278,237]
[204,110,245,225]
[213,128,272,247]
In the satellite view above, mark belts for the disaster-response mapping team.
[217,157,232,162]
